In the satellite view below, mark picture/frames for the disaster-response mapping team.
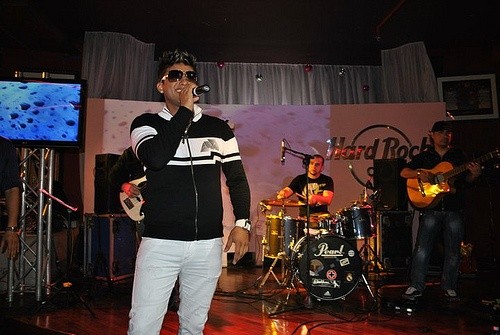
[437,74,499,120]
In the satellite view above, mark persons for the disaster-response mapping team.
[0,136,23,258]
[127,54,252,335]
[277,154,334,238]
[109,147,181,311]
[400,120,481,301]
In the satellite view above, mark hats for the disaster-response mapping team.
[430,122,454,142]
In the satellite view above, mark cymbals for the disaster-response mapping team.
[260,199,314,207]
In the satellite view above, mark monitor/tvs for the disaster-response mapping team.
[0,77,84,149]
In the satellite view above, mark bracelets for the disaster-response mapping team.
[6,226,18,232]
[416,169,421,178]
[234,219,251,231]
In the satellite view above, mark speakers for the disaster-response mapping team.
[95,154,129,214]
[378,214,412,269]
[373,159,408,211]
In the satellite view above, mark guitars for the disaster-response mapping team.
[119,175,148,222]
[406,147,500,212]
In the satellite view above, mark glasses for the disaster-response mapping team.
[159,70,198,82]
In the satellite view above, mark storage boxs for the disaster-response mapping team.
[85,213,138,281]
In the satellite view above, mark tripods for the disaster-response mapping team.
[258,149,382,324]
[30,210,96,318]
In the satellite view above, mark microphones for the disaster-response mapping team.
[280,141,285,165]
[191,84,210,97]
[280,140,285,164]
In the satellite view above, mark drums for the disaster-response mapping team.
[291,233,363,304]
[333,205,377,241]
[266,215,282,257]
[317,216,342,231]
[282,216,306,257]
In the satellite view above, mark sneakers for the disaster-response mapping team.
[439,290,459,301]
[402,286,423,300]
